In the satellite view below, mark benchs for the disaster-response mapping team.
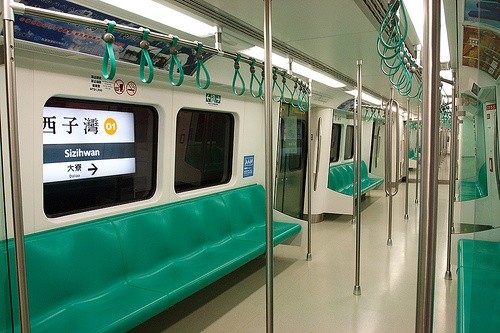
[455,238,500,333]
[459,157,488,225]
[327,159,384,199]
[409,147,417,169]
[0,182,302,333]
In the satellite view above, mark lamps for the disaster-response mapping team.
[399,1,458,116]
[344,88,415,120]
[99,0,221,40]
[237,45,348,89]
